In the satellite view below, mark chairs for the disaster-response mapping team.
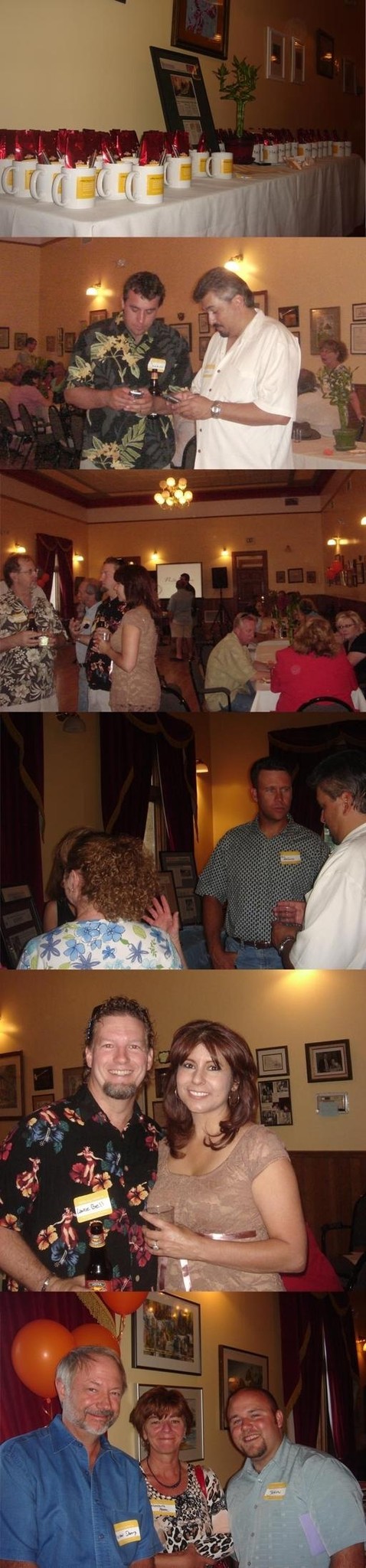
[155,665,192,712]
[199,643,215,678]
[66,413,86,469]
[318,1193,366,1292]
[191,625,210,656]
[0,397,46,469]
[188,659,232,712]
[47,404,75,469]
[17,403,56,469]
[294,695,356,713]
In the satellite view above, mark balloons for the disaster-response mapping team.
[11,1320,76,1405]
[70,1324,121,1360]
[100,1292,150,1319]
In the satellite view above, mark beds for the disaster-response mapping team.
[37,401,60,435]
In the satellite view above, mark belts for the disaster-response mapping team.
[231,937,274,950]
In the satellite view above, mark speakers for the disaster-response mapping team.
[212,567,228,589]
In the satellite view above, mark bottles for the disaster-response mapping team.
[147,368,162,420]
[83,1221,115,1293]
[283,627,287,638]
[269,622,276,639]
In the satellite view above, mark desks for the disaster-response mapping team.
[0,150,366,237]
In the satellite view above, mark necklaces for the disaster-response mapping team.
[146,1456,183,1490]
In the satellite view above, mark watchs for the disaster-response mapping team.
[277,936,296,957]
[138,1018,309,1292]
[210,400,223,419]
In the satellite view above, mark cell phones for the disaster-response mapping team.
[128,390,142,399]
[162,394,180,404]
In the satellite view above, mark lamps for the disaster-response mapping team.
[152,476,195,511]
[72,550,83,562]
[14,543,26,554]
[54,712,87,733]
[196,757,210,775]
[327,535,339,547]
[150,547,161,559]
[85,281,104,296]
[224,252,246,271]
[221,548,230,557]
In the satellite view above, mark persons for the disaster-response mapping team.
[69,1077,80,1096]
[316,338,366,428]
[1,553,366,713]
[161,264,304,469]
[269,748,366,970]
[1,993,165,1292]
[63,268,194,470]
[12,827,193,970]
[0,1345,166,1568]
[143,1300,194,1363]
[258,1081,291,1126]
[222,1386,366,1568]
[41,827,95,934]
[127,1384,237,1567]
[317,1052,331,1073]
[193,754,333,969]
[294,368,342,438]
[329,1058,342,1072]
[0,336,70,439]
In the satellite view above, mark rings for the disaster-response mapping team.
[152,1241,160,1250]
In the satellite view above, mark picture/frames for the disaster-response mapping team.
[197,311,211,334]
[157,869,184,932]
[276,570,286,583]
[132,1082,148,1117]
[290,330,301,346]
[303,1038,354,1084]
[306,570,317,584]
[343,55,358,95]
[288,568,304,584]
[169,0,232,62]
[217,1343,269,1431]
[257,1078,295,1127]
[45,308,108,358]
[130,1291,203,1377]
[13,331,28,351]
[308,305,342,356]
[325,553,366,588]
[151,1100,171,1130]
[0,1049,26,1122]
[350,302,366,322]
[254,1044,291,1079]
[153,1066,172,1099]
[132,1382,205,1464]
[158,850,203,927]
[198,336,211,362]
[168,321,192,354]
[315,27,337,80]
[60,1065,87,1099]
[251,289,268,316]
[0,881,44,970]
[290,34,307,88]
[277,305,300,328]
[0,326,9,349]
[265,25,287,83]
[31,1093,55,1112]
[350,322,366,355]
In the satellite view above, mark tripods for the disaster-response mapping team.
[210,588,233,637]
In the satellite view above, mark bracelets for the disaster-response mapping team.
[41,1271,56,1292]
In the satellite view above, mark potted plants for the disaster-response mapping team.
[315,363,361,452]
[212,54,263,166]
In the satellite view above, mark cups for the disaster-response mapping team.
[253,139,353,165]
[93,154,165,205]
[38,626,49,646]
[205,151,234,181]
[165,147,210,190]
[293,427,302,443]
[96,630,111,643]
[277,906,297,924]
[0,157,96,210]
[144,1203,177,1234]
